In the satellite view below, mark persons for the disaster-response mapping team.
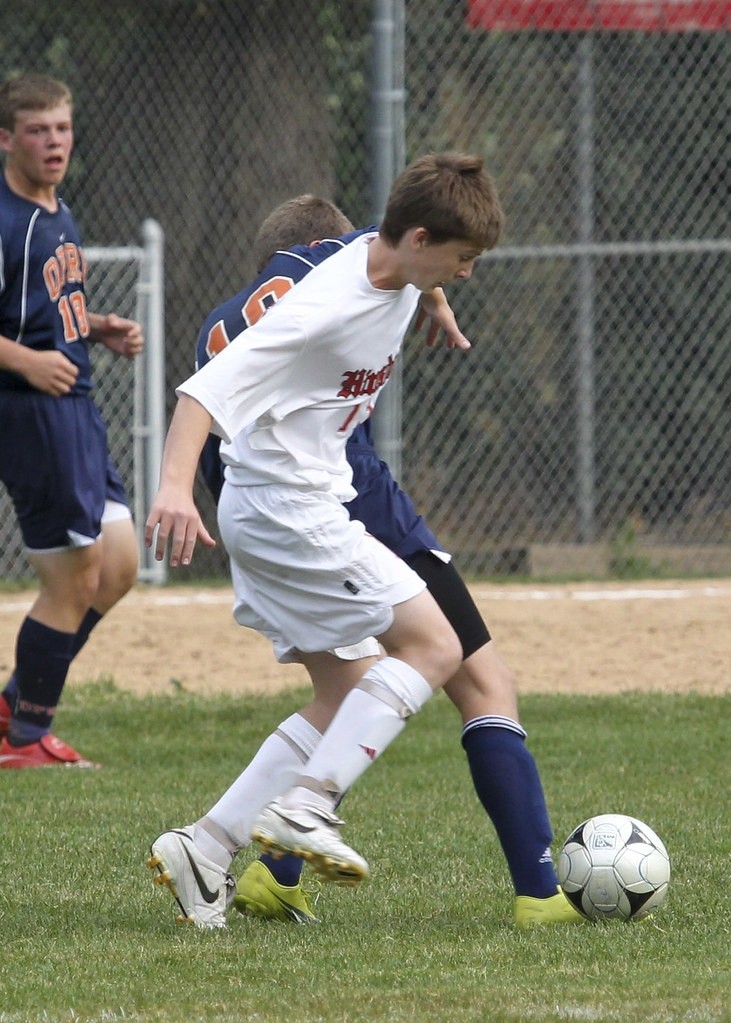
[0,71,148,772]
[192,195,585,928]
[144,150,505,930]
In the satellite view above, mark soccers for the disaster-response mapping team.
[558,812,670,924]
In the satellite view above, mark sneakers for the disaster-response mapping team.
[147,826,229,931]
[1,688,101,769]
[511,884,583,928]
[253,782,372,885]
[233,861,321,925]
